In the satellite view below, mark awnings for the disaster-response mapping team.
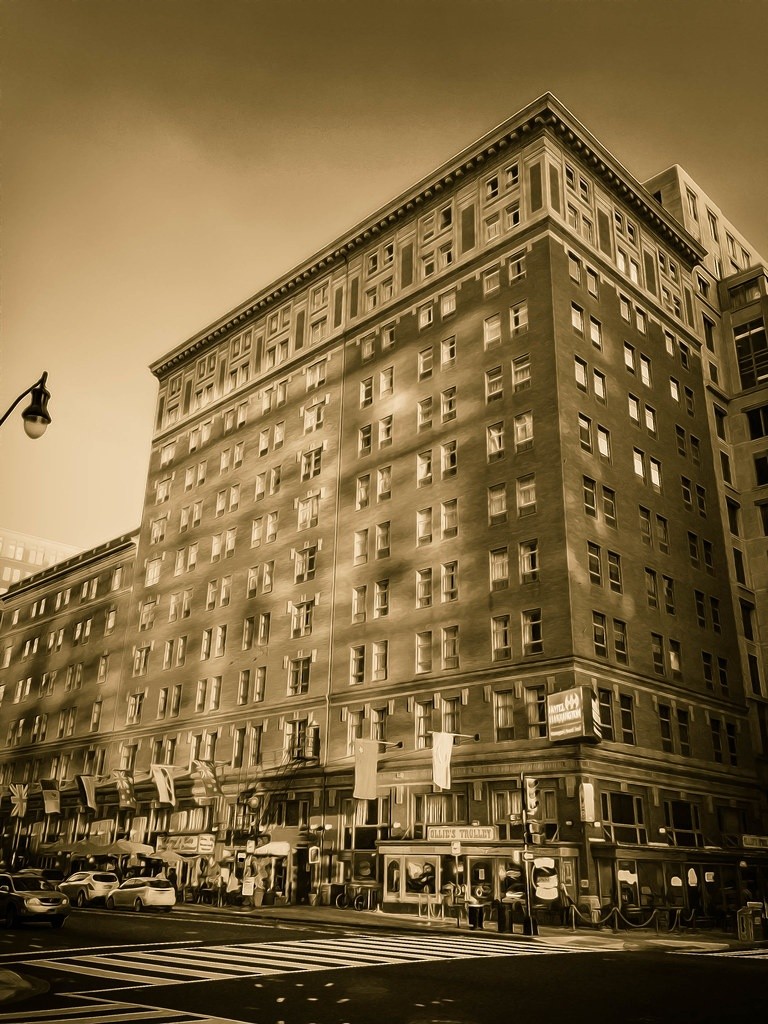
[254,841,296,856]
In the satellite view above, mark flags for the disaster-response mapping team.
[39,778,60,814]
[432,731,454,789]
[151,765,176,806]
[80,775,97,811]
[8,782,28,817]
[113,769,137,809]
[352,739,378,800]
[192,759,226,797]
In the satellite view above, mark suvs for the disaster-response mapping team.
[0,871,72,930]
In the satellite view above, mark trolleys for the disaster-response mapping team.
[335,880,367,911]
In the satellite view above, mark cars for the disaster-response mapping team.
[105,876,177,912]
[20,869,65,892]
[57,870,120,908]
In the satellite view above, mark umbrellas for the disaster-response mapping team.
[147,849,188,862]
[43,838,153,855]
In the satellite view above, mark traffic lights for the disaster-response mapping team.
[525,776,539,813]
[527,822,544,847]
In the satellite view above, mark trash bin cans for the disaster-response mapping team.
[468,903,485,931]
[497,901,513,933]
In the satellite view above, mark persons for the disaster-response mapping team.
[167,868,177,888]
